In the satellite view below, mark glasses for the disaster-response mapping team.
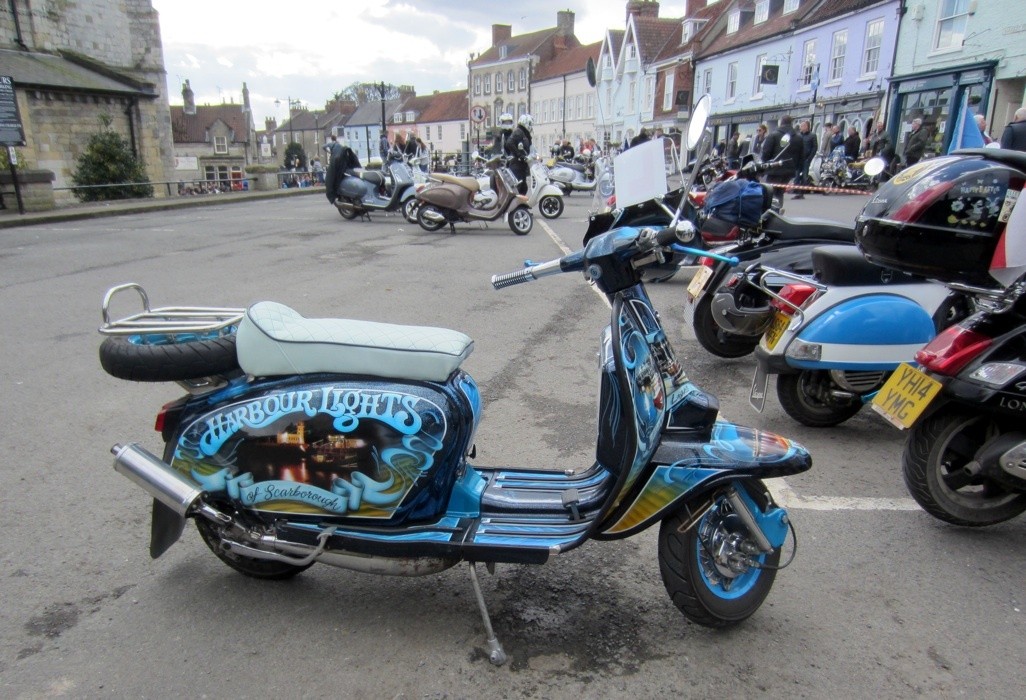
[756,129,762,132]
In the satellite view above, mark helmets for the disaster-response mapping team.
[499,113,513,129]
[517,114,534,133]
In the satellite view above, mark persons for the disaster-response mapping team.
[279,157,325,187]
[550,138,602,159]
[1000,106,1026,151]
[484,113,534,195]
[975,115,993,145]
[322,135,342,159]
[178,177,248,194]
[629,115,929,206]
[378,129,429,175]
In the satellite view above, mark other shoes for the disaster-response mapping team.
[791,194,805,199]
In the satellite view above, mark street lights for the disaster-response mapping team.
[274,96,301,144]
[314,109,320,156]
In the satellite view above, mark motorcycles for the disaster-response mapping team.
[743,245,1005,428]
[473,142,565,219]
[324,149,425,224]
[547,144,614,198]
[96,92,814,664]
[686,182,858,359]
[857,149,1026,527]
[795,146,877,196]
[581,133,793,284]
[407,133,534,235]
[384,145,428,196]
[688,142,727,186]
